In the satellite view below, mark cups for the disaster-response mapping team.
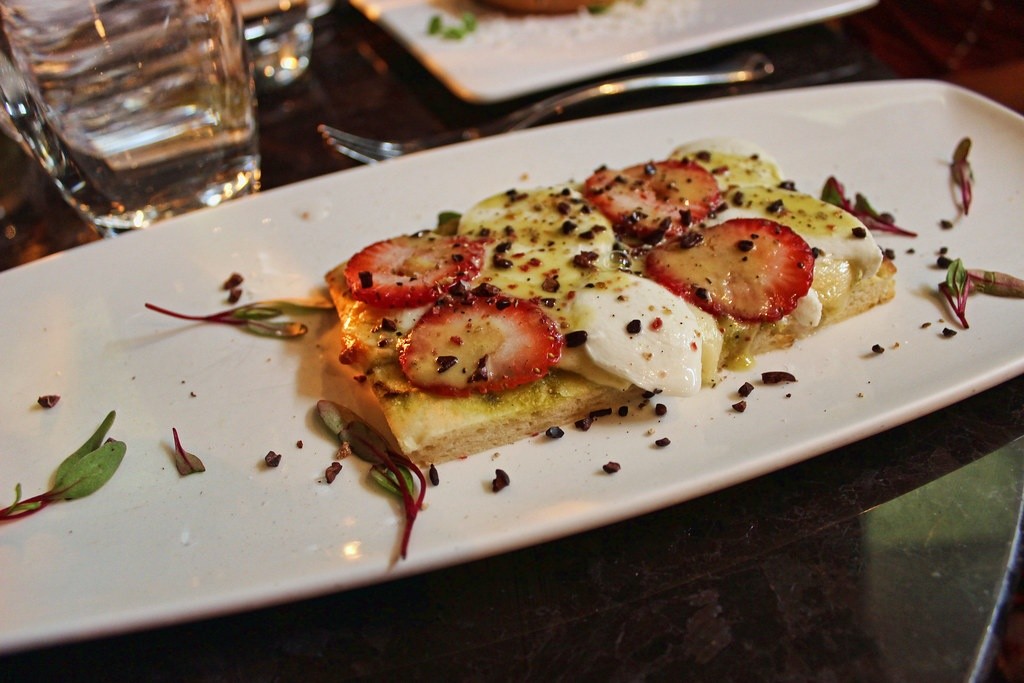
[239,0,314,89]
[0,0,257,237]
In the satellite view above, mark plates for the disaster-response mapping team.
[0,77,1024,655]
[345,0,878,103]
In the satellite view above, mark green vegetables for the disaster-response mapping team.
[822,138,1024,331]
[0,297,426,560]
[429,8,476,39]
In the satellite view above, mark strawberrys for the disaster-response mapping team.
[585,155,723,237]
[396,289,562,396]
[645,216,814,323]
[340,229,486,306]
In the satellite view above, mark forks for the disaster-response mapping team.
[318,70,751,165]
